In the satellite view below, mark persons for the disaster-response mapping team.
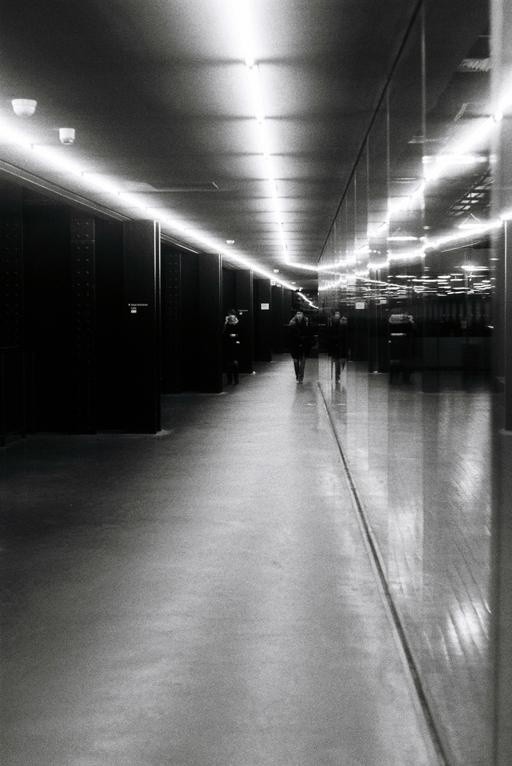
[223,314,241,386]
[287,310,313,382]
[323,311,350,385]
